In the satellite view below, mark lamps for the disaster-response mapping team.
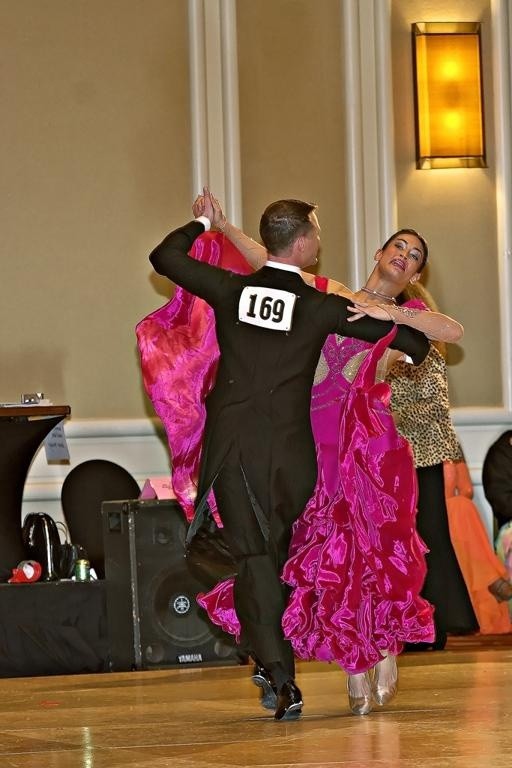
[410,21,490,171]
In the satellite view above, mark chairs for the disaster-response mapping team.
[62,459,141,579]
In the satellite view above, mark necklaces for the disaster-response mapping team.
[360,286,395,301]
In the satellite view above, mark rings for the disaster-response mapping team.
[201,204,205,207]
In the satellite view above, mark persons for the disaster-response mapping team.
[147,186,432,720]
[482,427,512,574]
[385,278,481,651]
[134,195,466,715]
[443,442,512,635]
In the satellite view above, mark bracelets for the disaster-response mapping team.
[216,214,226,229]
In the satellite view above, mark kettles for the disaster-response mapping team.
[20,512,63,582]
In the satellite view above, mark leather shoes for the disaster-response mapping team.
[343,669,374,715]
[372,653,400,707]
[251,665,277,709]
[268,680,303,722]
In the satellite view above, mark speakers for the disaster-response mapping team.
[101,497,251,673]
